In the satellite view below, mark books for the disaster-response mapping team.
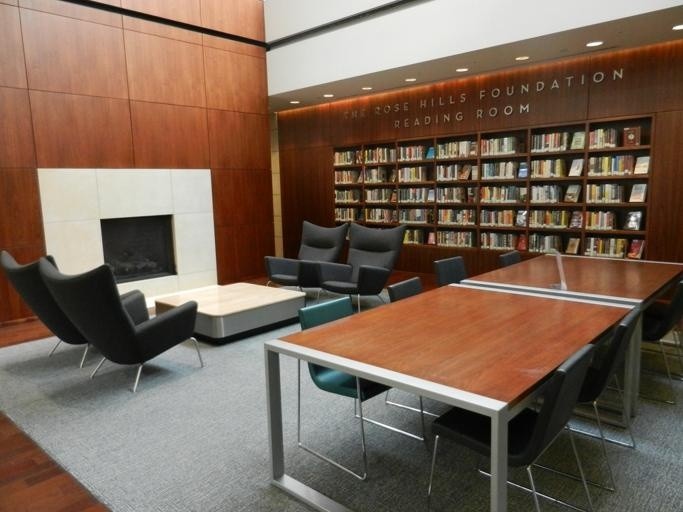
[333,146,396,222]
[399,137,528,251]
[528,128,650,259]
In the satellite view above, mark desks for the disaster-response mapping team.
[265,280,639,512]
[461,261,682,417]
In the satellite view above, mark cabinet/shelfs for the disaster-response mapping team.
[332,114,656,260]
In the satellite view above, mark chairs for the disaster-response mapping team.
[434,255,469,288]
[382,276,442,420]
[427,343,596,512]
[519,296,643,492]
[263,219,407,314]
[500,251,523,268]
[0,249,204,396]
[605,272,682,406]
[299,296,427,481]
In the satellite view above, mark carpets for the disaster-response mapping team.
[1,321,683,511]
